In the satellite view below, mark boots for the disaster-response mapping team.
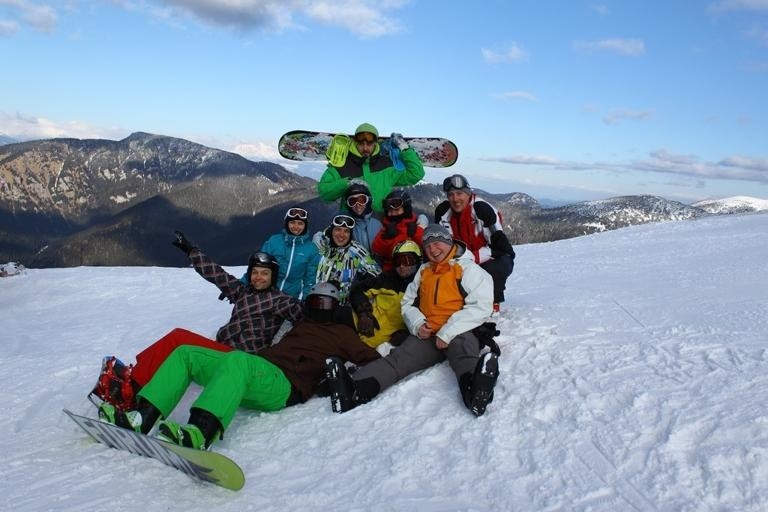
[97,356,158,437]
[158,410,223,451]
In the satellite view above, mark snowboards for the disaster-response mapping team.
[279,130,460,169]
[64,400,245,492]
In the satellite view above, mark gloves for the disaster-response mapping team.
[356,310,380,337]
[172,229,196,254]
[390,132,409,152]
[312,231,325,249]
[350,180,369,187]
[389,329,410,347]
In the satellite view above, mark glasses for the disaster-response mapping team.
[332,215,355,228]
[382,197,403,210]
[346,194,372,207]
[443,174,471,190]
[283,207,308,221]
[355,131,378,143]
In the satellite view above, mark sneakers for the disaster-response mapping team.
[491,303,500,317]
[470,351,498,415]
[331,358,357,413]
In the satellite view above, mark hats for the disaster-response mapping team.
[247,251,280,287]
[443,176,472,196]
[354,123,378,143]
[329,215,353,245]
[423,223,453,250]
[285,207,308,236]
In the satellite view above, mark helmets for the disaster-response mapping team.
[382,190,412,221]
[345,185,372,218]
[391,239,423,267]
[304,282,341,323]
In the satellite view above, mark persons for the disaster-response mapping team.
[371,189,424,269]
[318,121,425,221]
[312,184,383,251]
[218,207,322,304]
[96,230,304,410]
[316,215,382,307]
[98,283,381,449]
[434,175,515,315]
[327,225,500,417]
[336,239,424,349]
